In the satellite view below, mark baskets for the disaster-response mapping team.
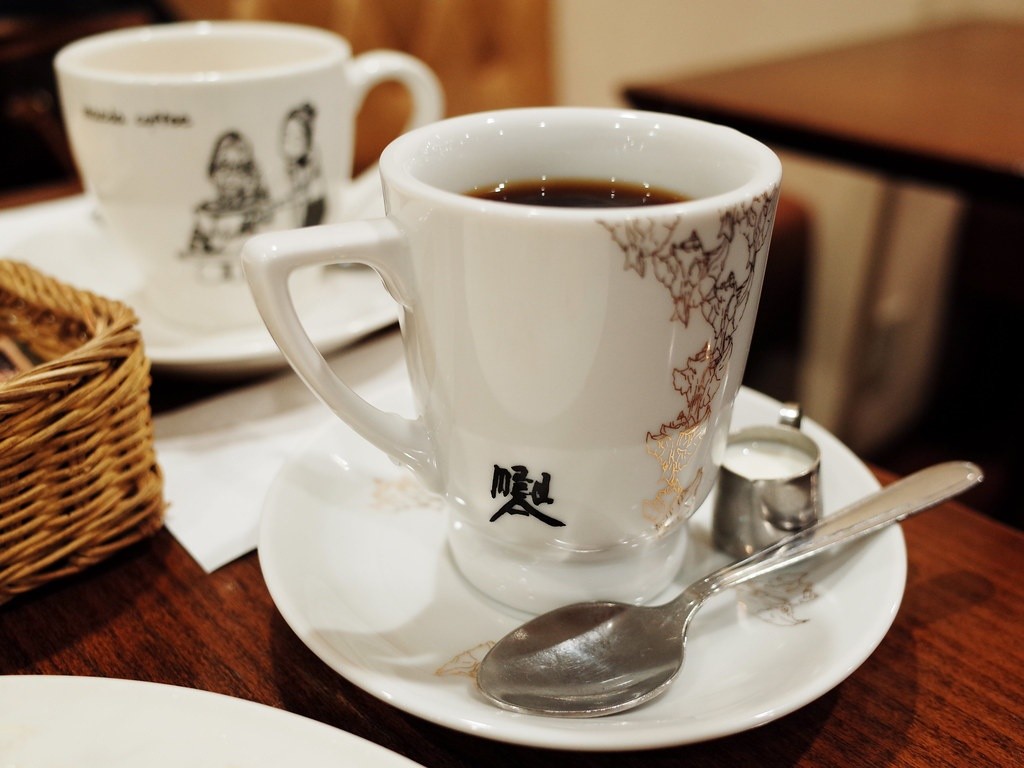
[0,260,162,603]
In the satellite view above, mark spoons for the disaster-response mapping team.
[475,461,983,718]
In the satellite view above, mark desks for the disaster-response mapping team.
[620,13,1023,217]
[0,186,1024,767]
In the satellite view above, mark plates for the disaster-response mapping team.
[259,387,907,750]
[1,674,427,768]
[2,194,399,371]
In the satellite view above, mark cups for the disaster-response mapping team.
[55,21,446,311]
[243,107,781,615]
[712,403,824,560]
